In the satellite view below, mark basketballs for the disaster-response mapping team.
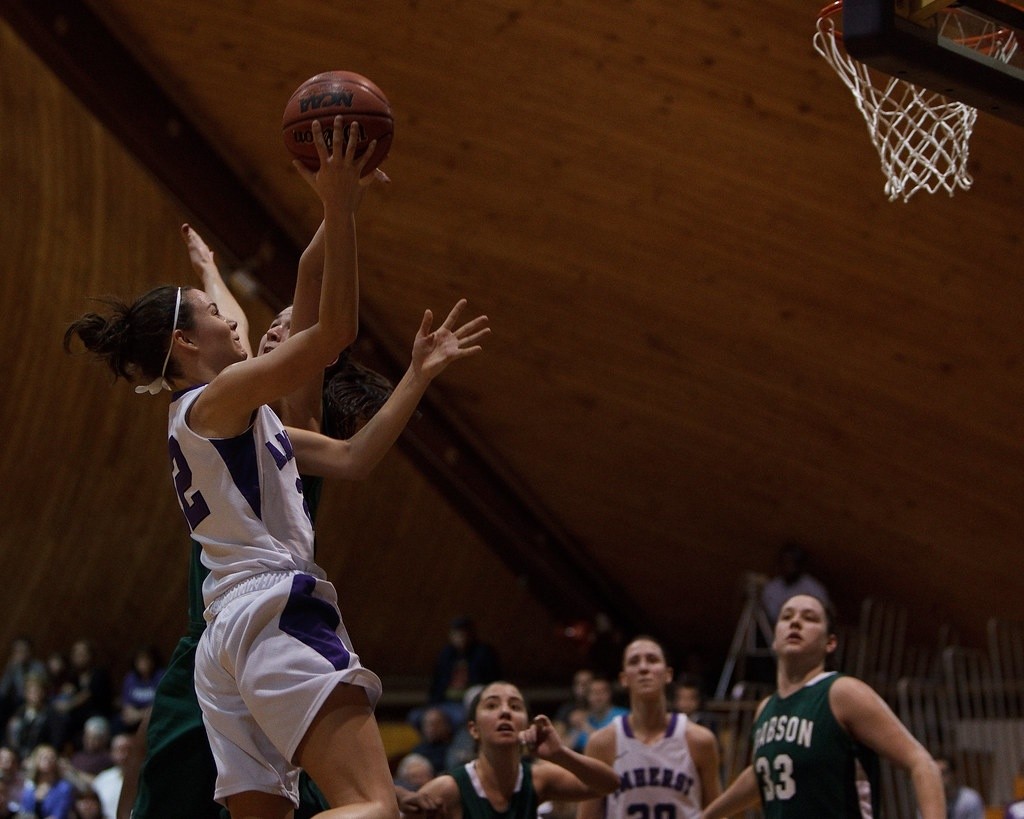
[281,69,394,177]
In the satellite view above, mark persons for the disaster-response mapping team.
[1,637,167,819]
[674,682,716,738]
[698,588,948,819]
[64,115,490,818]
[575,640,727,819]
[402,679,620,819]
[396,613,630,819]
[915,762,983,819]
